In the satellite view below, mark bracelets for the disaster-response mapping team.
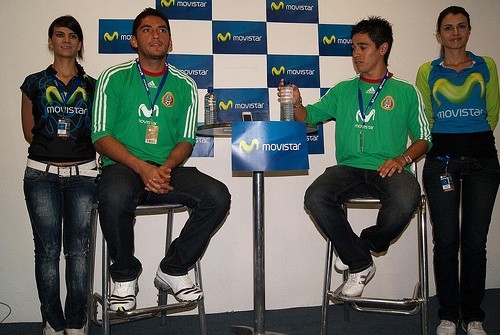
[400,153,413,166]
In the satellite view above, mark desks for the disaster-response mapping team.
[196,123,318,335]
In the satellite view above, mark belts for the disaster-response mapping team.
[26,159,99,177]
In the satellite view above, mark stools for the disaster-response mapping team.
[84,152,207,335]
[320,161,429,335]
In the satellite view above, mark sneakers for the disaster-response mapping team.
[437,320,457,335]
[466,321,486,335]
[153,265,204,304]
[336,257,348,270]
[341,262,375,296]
[107,276,139,313]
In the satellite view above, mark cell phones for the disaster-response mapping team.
[242,111,253,122]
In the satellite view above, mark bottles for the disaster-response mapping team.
[279,80,294,122]
[204,87,217,124]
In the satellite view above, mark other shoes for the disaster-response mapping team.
[66,325,86,335]
[43,322,64,335]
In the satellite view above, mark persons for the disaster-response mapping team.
[21,15,97,335]
[277,16,432,297]
[416,6,500,335]
[92,6,231,312]
